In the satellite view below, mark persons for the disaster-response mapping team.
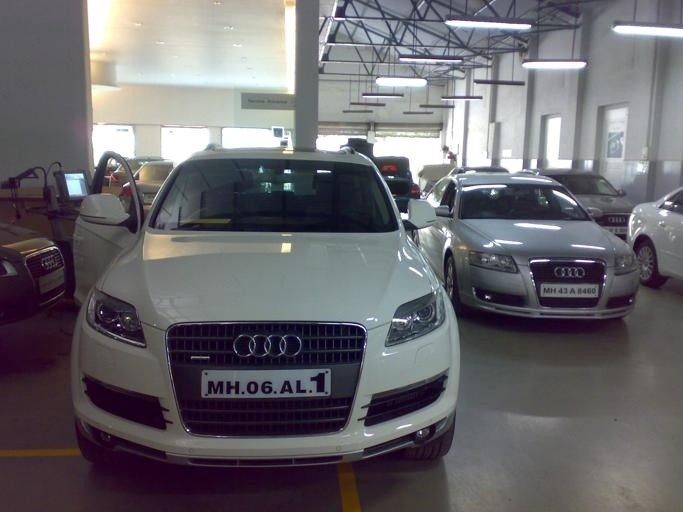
[279,136,289,147]
[441,144,457,166]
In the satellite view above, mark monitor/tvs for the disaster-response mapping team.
[56,169,89,201]
[272,126,284,139]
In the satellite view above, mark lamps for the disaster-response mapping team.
[419,65,455,108]
[341,75,373,113]
[611,3,682,40]
[444,1,532,31]
[440,60,483,100]
[403,88,432,114]
[361,80,404,99]
[350,66,388,106]
[398,2,463,63]
[522,2,587,71]
[472,31,526,85]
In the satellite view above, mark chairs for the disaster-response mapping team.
[462,188,485,219]
[198,172,352,216]
